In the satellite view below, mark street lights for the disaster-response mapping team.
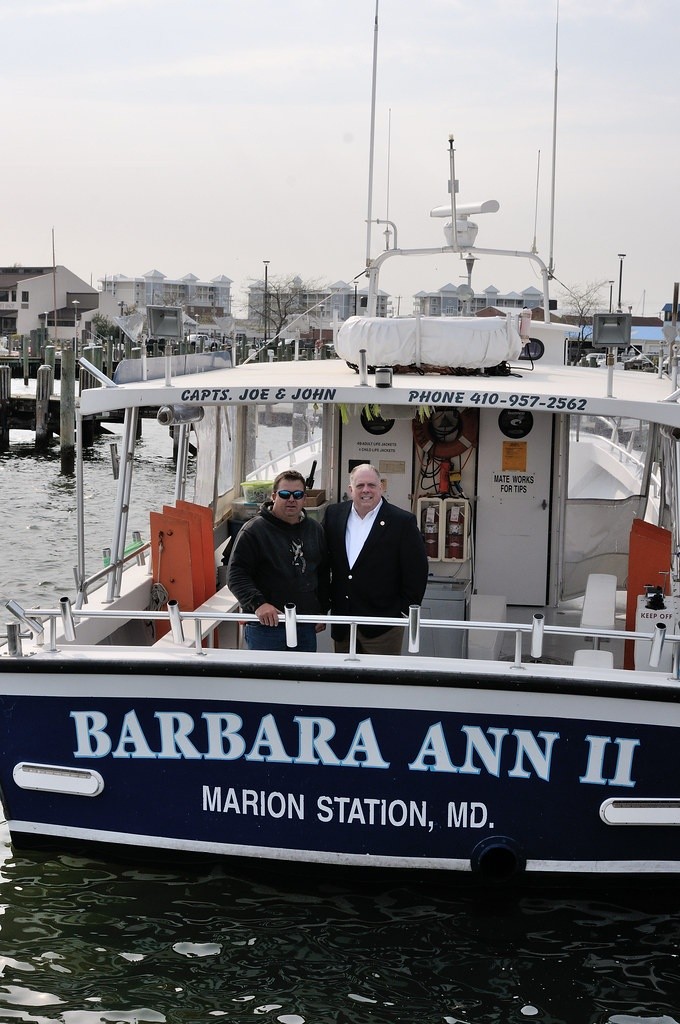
[608,280,616,312]
[71,299,80,364]
[42,311,49,329]
[352,280,359,317]
[615,252,626,311]
[262,260,271,342]
[194,313,199,335]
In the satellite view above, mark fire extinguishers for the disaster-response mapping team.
[444,503,466,558]
[421,504,440,559]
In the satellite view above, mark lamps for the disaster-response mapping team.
[592,314,632,396]
[146,304,183,384]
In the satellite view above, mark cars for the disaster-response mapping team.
[185,333,221,352]
[278,338,304,351]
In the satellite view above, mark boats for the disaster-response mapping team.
[0,0,678,898]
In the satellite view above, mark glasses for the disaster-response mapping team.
[274,489,306,500]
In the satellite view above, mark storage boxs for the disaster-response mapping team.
[230,497,264,522]
[303,489,327,506]
[240,480,274,503]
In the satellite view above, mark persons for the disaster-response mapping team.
[319,463,428,655]
[226,471,330,652]
